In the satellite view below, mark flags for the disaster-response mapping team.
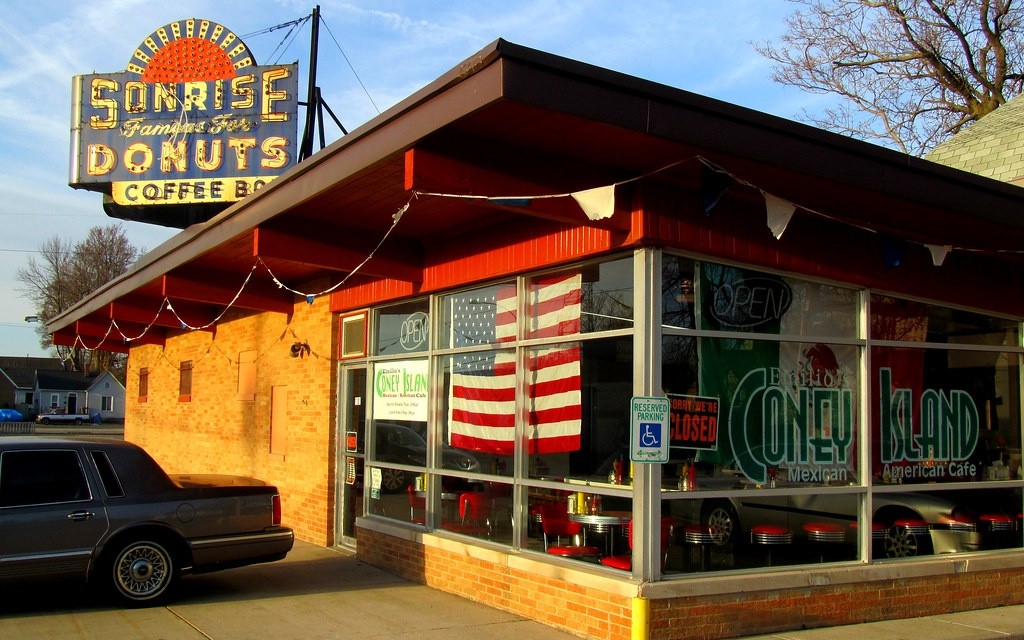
[692,259,928,478]
[442,272,584,456]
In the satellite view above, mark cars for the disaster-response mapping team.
[666,444,980,554]
[356,423,481,492]
[0,434,294,604]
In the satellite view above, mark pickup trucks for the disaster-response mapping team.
[36,407,89,425]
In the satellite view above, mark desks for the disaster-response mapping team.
[569,512,632,561]
[415,491,458,501]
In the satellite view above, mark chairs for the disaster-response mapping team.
[540,499,600,558]
[408,484,426,524]
[440,492,493,534]
[601,516,677,570]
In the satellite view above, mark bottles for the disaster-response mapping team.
[884,468,903,485]
[678,458,696,492]
[578,491,597,515]
[609,458,622,485]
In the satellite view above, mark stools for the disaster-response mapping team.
[750,526,792,567]
[978,515,1013,534]
[893,519,931,535]
[929,517,975,532]
[851,522,890,540]
[803,522,846,562]
[685,525,728,571]
